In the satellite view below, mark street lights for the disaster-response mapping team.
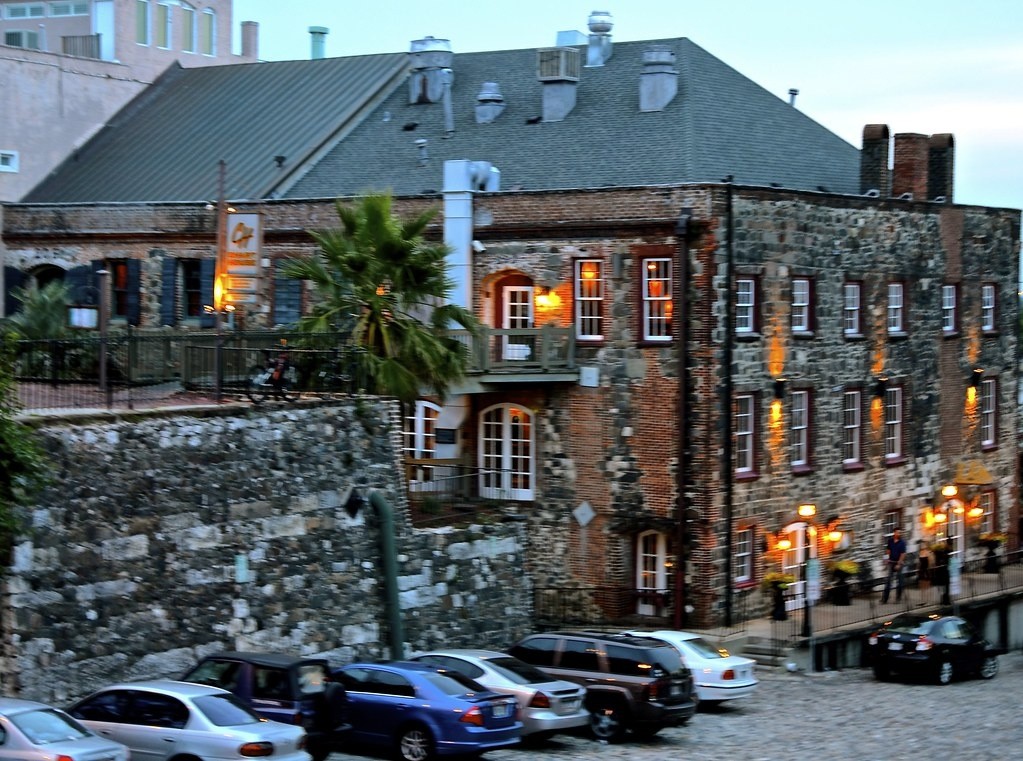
[926,487,984,605]
[777,503,842,637]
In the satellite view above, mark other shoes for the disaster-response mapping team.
[879,600,887,603]
[894,599,900,603]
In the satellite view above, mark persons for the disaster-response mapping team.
[916,539,933,606]
[878,527,907,603]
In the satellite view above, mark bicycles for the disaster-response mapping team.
[246,337,355,404]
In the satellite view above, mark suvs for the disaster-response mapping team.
[501,630,699,743]
[177,651,352,761]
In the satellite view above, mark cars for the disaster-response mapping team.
[58,680,313,761]
[864,611,999,686]
[406,648,591,741]
[619,630,760,713]
[0,697,131,761]
[331,660,524,761]
